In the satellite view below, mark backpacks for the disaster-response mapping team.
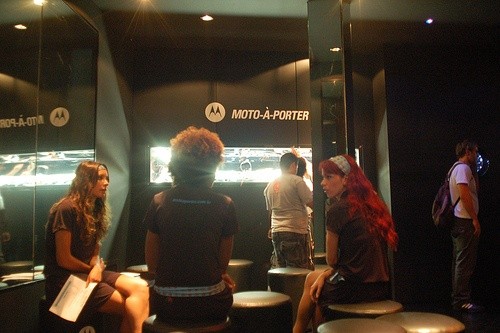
[432,162,473,232]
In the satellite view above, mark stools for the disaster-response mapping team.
[0,259,33,275]
[40,255,468,333]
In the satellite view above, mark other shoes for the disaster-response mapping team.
[449,303,487,313]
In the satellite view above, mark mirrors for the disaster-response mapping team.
[0,0,100,294]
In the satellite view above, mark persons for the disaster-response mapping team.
[42,161,149,333]
[291,154,399,333]
[448,137,484,312]
[148,127,237,324]
[263,152,317,271]
